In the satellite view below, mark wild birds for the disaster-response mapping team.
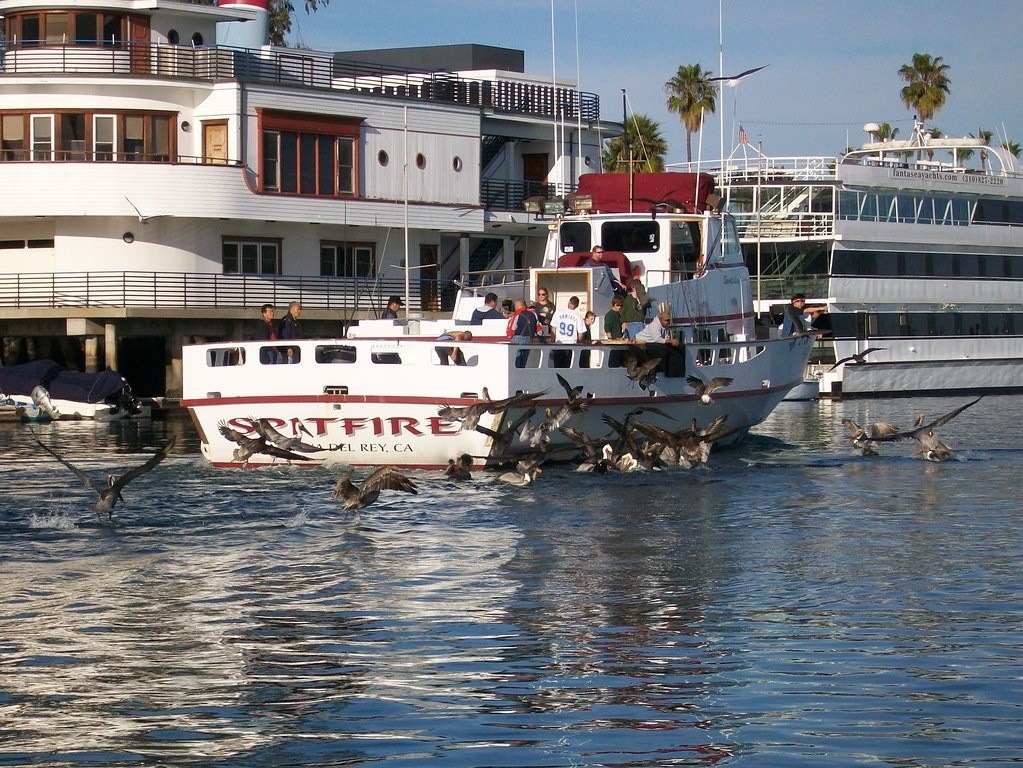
[216,415,344,469]
[28,424,177,524]
[828,347,891,372]
[330,465,420,513]
[840,395,985,462]
[705,64,770,88]
[437,345,734,487]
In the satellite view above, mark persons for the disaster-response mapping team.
[549,296,597,369]
[780,293,808,337]
[382,296,406,319]
[630,304,680,365]
[282,302,304,364]
[604,294,626,367]
[256,304,286,364]
[471,292,507,326]
[536,288,557,335]
[433,331,473,367]
[584,245,628,297]
[620,276,647,367]
[506,298,537,368]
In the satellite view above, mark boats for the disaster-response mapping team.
[178,190,825,470]
[705,121,1022,403]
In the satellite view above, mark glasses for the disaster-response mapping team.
[594,251,603,254]
[615,304,623,307]
[538,293,546,296]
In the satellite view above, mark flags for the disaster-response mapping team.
[740,123,751,144]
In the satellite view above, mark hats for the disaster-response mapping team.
[660,312,671,320]
[389,295,406,306]
[525,301,536,308]
